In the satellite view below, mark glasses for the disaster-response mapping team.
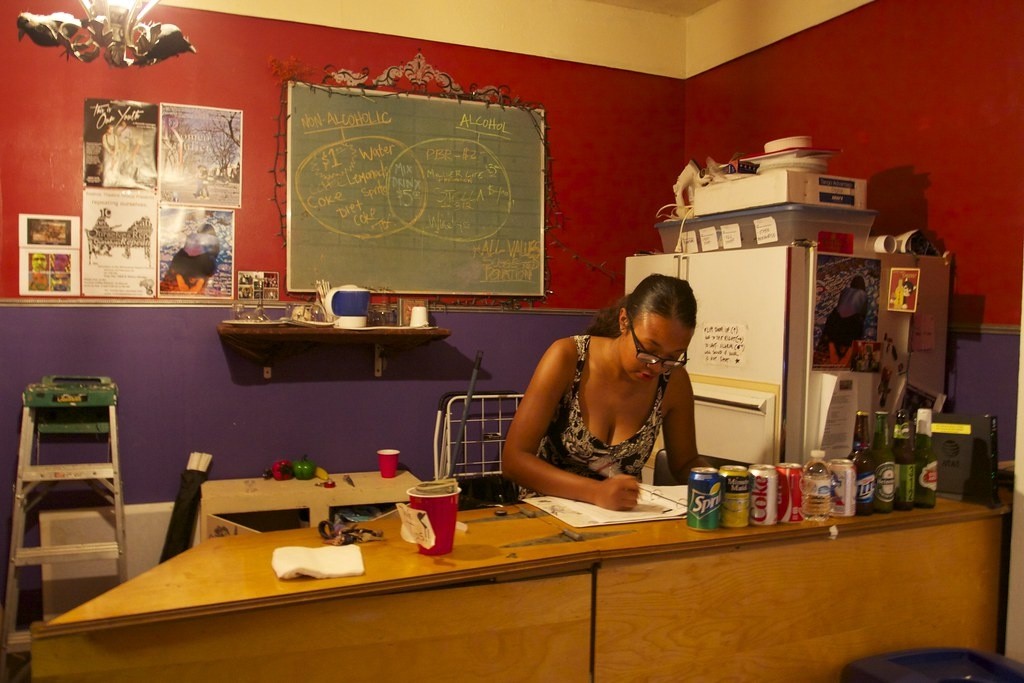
[630,318,687,369]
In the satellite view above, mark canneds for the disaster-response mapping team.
[774,463,804,524]
[685,467,721,531]
[746,463,779,526]
[825,459,856,517]
[718,465,751,528]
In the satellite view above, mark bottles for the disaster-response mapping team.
[872,412,895,514]
[847,411,874,515]
[893,409,916,510]
[914,408,937,509]
[802,450,831,522]
[234,273,400,330]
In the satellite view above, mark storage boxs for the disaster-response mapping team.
[653,204,878,255]
[694,168,868,218]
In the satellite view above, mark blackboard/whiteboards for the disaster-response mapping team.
[285,80,544,297]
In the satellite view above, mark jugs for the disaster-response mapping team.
[325,284,370,329]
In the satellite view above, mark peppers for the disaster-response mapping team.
[271,453,316,480]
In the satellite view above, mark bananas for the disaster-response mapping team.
[314,467,329,480]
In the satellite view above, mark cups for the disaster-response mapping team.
[406,488,462,556]
[376,449,400,478]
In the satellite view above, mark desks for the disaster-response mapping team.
[30,485,1015,683]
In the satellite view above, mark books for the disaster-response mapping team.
[518,483,690,528]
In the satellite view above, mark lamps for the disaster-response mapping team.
[16,0,198,69]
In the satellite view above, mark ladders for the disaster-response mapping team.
[0,374,128,683]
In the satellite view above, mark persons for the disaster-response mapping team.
[29,254,71,291]
[500,274,715,512]
[821,274,868,359]
[170,223,220,292]
[101,120,148,190]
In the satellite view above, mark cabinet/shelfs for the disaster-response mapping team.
[199,471,422,541]
